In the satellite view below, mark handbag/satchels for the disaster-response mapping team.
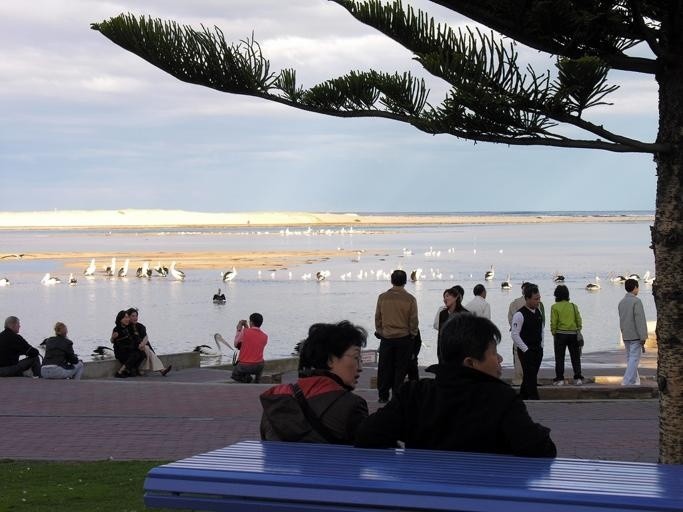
[576,329,585,347]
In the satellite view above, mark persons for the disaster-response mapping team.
[258,320,369,445]
[111,309,145,377]
[0,316,40,378]
[431,281,584,401]
[351,313,559,461]
[372,269,418,403]
[109,306,172,376]
[38,321,84,380]
[618,278,648,388]
[230,312,267,384]
[405,328,422,381]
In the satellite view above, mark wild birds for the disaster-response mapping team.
[501,272,513,289]
[223,264,237,283]
[584,269,658,292]
[551,271,566,282]
[0,278,10,286]
[211,288,226,302]
[69,272,77,285]
[484,263,495,280]
[82,256,185,283]
[0,254,36,264]
[41,272,61,287]
[193,333,234,356]
[256,265,464,282]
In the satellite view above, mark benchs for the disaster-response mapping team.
[144,439,682,511]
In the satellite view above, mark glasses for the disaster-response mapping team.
[339,351,363,362]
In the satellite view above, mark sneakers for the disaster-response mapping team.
[160,364,172,377]
[113,370,136,378]
[573,377,583,386]
[550,379,565,386]
[376,392,389,403]
